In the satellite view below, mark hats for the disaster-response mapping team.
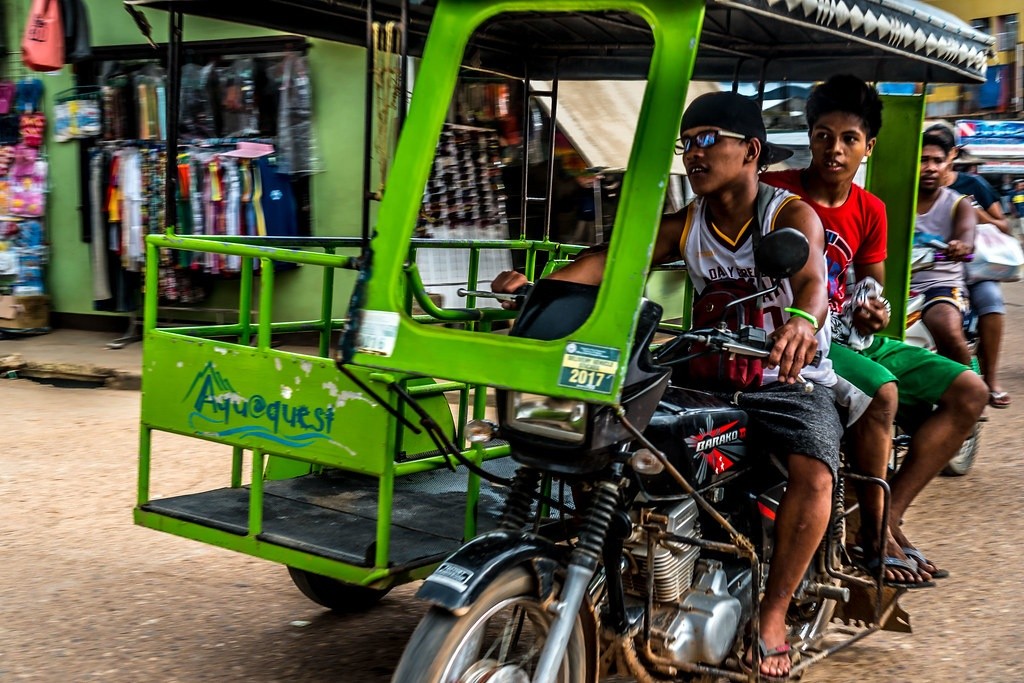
[681,91,794,165]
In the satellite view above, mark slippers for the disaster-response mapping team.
[988,390,1012,409]
[743,634,791,683]
[902,548,950,578]
[855,555,938,588]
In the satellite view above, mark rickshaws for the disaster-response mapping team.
[127,0,997,683]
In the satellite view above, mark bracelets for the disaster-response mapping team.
[783,306,819,333]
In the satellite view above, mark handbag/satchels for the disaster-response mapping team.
[688,278,762,389]
[21,0,65,72]
[967,224,1023,282]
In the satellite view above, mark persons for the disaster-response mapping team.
[489,91,843,680]
[908,123,1013,421]
[758,76,993,588]
[998,173,1024,241]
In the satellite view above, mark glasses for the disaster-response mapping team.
[674,130,751,156]
